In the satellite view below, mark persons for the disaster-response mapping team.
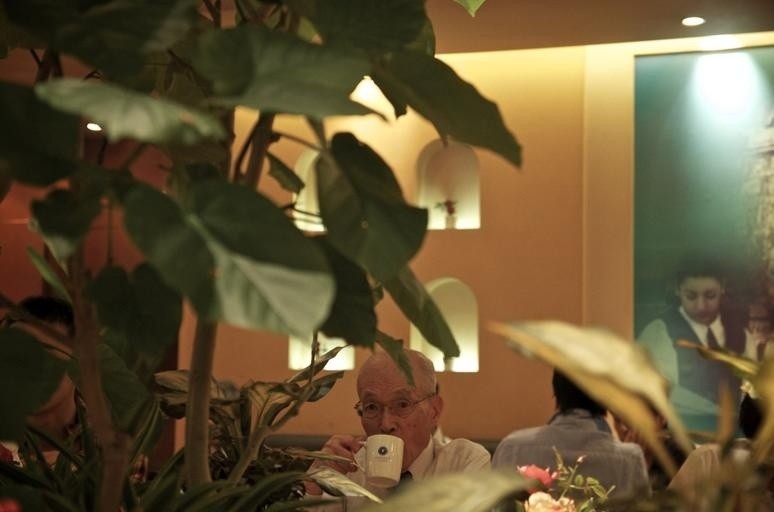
[494,366,655,502]
[614,389,773,511]
[635,258,744,443]
[1,294,152,512]
[745,294,774,397]
[297,347,492,512]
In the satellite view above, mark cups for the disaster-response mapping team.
[352,433,404,488]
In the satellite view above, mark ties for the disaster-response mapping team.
[705,328,719,354]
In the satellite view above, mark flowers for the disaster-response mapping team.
[510,440,617,512]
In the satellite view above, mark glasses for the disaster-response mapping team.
[352,391,438,419]
